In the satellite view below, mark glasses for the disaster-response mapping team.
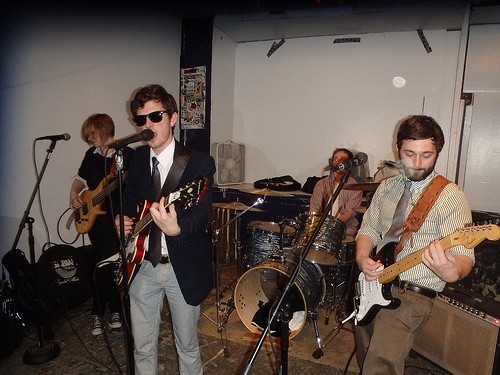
[132,110,168,127]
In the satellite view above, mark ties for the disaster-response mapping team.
[385,180,412,239]
[147,157,162,268]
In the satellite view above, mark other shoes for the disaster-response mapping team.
[336,295,344,304]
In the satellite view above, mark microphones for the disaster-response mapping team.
[110,129,154,148]
[36,133,71,141]
[332,152,367,171]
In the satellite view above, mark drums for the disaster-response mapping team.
[243,220,297,269]
[339,235,357,264]
[289,211,347,265]
[233,248,327,340]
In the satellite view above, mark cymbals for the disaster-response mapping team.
[351,206,368,213]
[340,181,381,191]
[211,202,267,212]
[238,188,295,197]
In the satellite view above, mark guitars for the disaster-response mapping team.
[344,219,500,327]
[112,176,209,286]
[74,170,129,234]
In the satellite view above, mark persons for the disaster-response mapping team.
[309,148,362,237]
[70,113,136,336]
[109,84,216,375]
[351,114,475,375]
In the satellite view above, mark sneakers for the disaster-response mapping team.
[92,315,106,335]
[109,313,122,329]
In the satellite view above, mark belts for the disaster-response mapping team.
[144,251,170,264]
[394,280,437,299]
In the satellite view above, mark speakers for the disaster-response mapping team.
[413,284,500,375]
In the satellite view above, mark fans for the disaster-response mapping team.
[211,140,245,186]
